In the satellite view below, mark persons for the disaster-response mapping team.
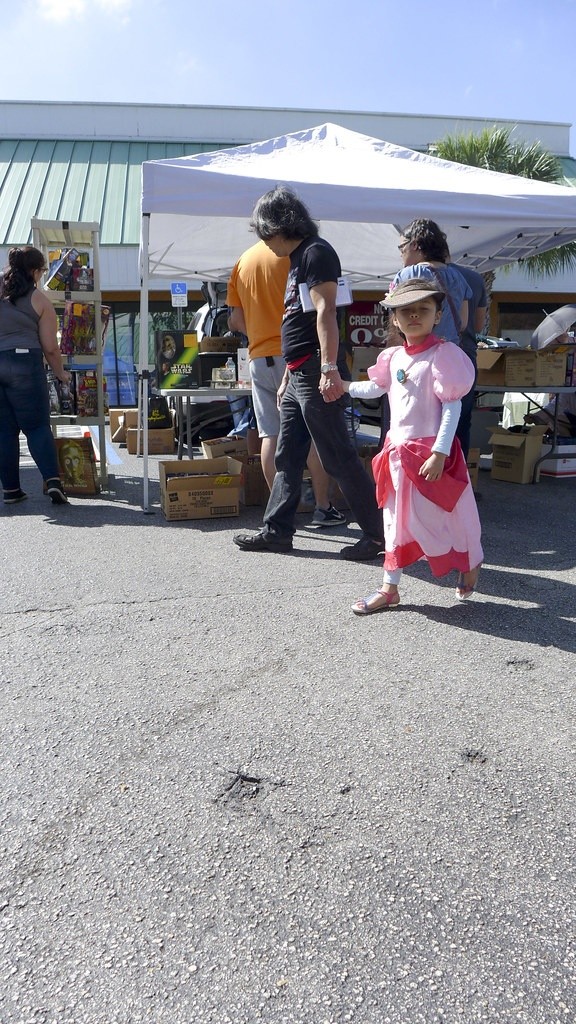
[234,186,385,561]
[318,278,484,613]
[548,325,576,402]
[448,261,486,462]
[226,240,347,525]
[390,218,473,347]
[0,246,69,506]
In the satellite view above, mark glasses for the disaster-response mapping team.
[398,240,413,254]
[32,268,48,273]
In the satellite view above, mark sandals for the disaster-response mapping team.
[455,561,479,602]
[233,532,294,554]
[351,590,399,614]
[340,535,385,560]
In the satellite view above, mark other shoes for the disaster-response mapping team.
[474,492,482,501]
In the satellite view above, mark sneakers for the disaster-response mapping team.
[2,488,28,504]
[311,500,346,525]
[46,480,68,505]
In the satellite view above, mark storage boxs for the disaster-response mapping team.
[59,298,111,356]
[538,443,576,478]
[43,247,80,291]
[199,336,242,353]
[69,268,94,292]
[200,435,246,459]
[41,436,99,495]
[124,426,175,454]
[485,423,551,484]
[537,344,574,387]
[158,456,243,520]
[228,449,271,506]
[75,375,109,416]
[474,349,539,386]
[109,408,140,442]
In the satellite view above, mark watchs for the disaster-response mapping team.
[320,364,338,373]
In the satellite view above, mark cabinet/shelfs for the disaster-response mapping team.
[31,215,105,490]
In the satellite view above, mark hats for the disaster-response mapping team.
[379,279,446,311]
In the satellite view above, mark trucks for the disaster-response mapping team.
[144,279,389,443]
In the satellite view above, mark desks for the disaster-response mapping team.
[160,387,364,461]
[472,384,575,484]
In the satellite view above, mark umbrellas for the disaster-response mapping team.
[531,303,576,349]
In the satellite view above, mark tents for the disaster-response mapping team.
[136,123,576,513]
